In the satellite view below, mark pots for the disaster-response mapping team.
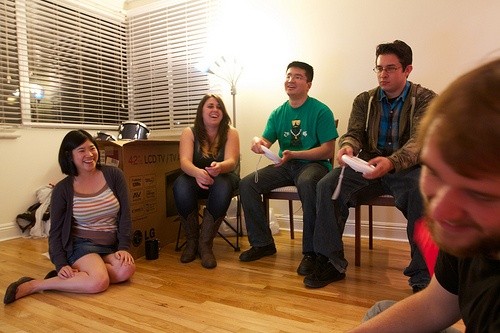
[118,121,149,140]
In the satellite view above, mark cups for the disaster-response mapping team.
[145,238,159,260]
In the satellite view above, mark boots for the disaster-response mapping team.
[181,207,198,263]
[198,208,227,268]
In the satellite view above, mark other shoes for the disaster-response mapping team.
[240,243,276,261]
[297,252,315,275]
[403,266,430,291]
[303,270,346,289]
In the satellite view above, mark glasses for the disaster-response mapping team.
[373,65,403,73]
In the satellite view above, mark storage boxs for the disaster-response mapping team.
[94,134,182,260]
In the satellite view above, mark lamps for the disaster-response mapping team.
[195,40,256,235]
[7,83,45,122]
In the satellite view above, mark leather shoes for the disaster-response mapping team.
[3,277,35,305]
[44,271,58,279]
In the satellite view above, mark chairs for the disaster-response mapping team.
[355,194,395,266]
[263,118,340,256]
[175,156,244,253]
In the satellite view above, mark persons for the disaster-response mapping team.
[239,61,339,277]
[174,94,240,269]
[4,129,135,303]
[342,58,500,333]
[303,40,440,294]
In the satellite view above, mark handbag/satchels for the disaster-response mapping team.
[15,202,51,233]
